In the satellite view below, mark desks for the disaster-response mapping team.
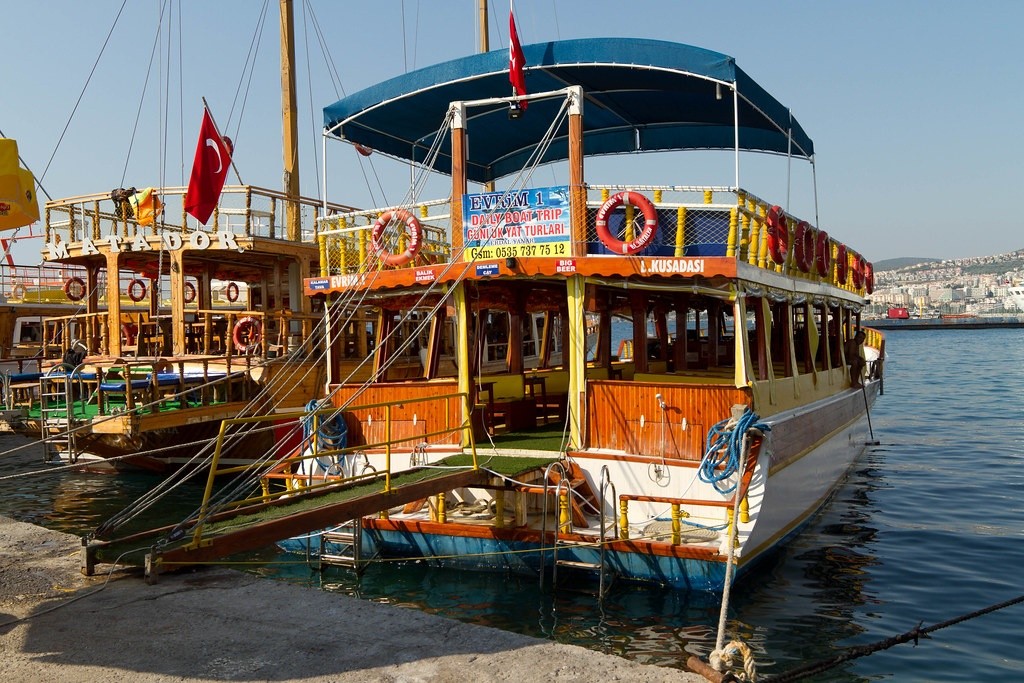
[9,383,47,410]
[150,315,172,356]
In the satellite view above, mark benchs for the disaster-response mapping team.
[99,372,243,415]
[428,346,834,435]
[96,312,162,355]
[51,374,97,401]
[2,373,43,398]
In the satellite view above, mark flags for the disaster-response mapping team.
[183,109,232,225]
[508,11,529,109]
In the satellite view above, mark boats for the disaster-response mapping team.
[0,234,264,434]
[264,38,885,601]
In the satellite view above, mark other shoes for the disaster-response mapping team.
[850,382,864,388]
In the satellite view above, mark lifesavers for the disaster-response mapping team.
[226,283,239,302]
[233,317,264,351]
[595,192,659,254]
[11,283,27,300]
[837,246,849,285]
[121,323,132,355]
[817,230,832,278]
[371,210,423,265]
[65,277,87,301]
[129,279,147,302]
[793,221,815,272]
[767,205,791,266]
[184,282,196,303]
[864,262,874,295]
[852,253,866,289]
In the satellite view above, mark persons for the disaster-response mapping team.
[62,339,87,372]
[843,330,867,390]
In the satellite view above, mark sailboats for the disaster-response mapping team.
[0,0,596,468]
